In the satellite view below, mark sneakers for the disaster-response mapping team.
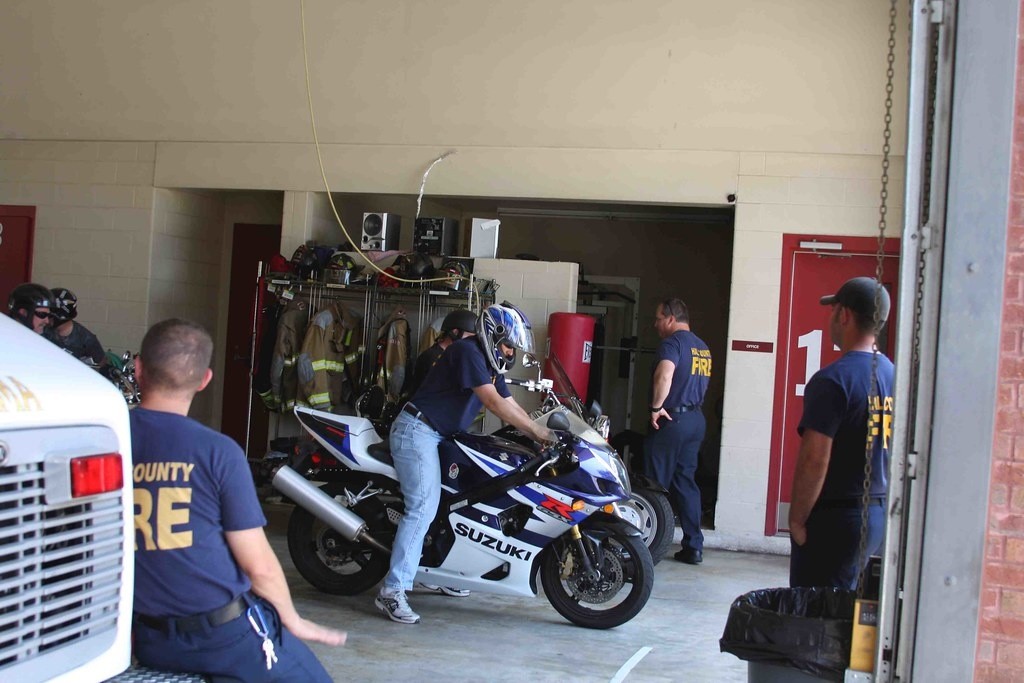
[375,586,420,624]
[419,581,470,596]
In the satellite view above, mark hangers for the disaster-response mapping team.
[333,296,343,312]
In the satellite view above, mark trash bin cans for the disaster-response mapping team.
[741,586,862,683]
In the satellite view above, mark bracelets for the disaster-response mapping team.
[652,406,662,412]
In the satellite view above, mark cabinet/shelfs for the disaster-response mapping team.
[242,258,500,500]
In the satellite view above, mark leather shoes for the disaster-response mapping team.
[674,548,702,563]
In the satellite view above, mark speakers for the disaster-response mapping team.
[463,218,499,259]
[361,212,402,252]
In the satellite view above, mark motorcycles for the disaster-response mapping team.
[262,352,675,630]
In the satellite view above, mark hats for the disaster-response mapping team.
[820,277,891,321]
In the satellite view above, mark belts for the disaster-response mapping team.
[816,497,885,507]
[136,590,252,631]
[404,404,436,431]
[666,404,701,413]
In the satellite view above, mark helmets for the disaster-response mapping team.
[441,309,478,333]
[48,287,77,328]
[7,283,57,329]
[475,300,531,374]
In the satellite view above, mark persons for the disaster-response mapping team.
[42,287,106,374]
[787,275,897,589]
[414,309,478,394]
[127,317,350,683]
[376,300,549,629]
[643,297,711,562]
[8,282,55,335]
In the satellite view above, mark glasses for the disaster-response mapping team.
[665,296,673,317]
[33,310,52,320]
